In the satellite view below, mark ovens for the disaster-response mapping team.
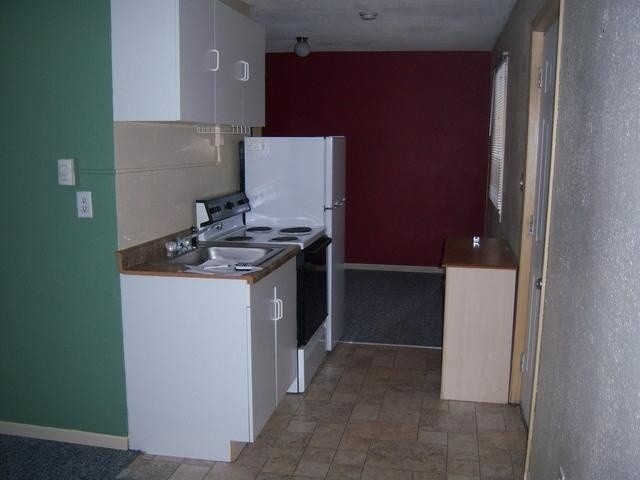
[297,235,333,347]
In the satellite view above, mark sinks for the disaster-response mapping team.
[170,244,269,267]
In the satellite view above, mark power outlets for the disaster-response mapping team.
[77,191,93,218]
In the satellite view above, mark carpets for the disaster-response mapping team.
[338,269,444,349]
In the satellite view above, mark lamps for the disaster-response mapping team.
[294,36,311,57]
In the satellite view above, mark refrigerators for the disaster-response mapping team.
[243,135,347,353]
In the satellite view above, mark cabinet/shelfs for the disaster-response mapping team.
[120,256,299,463]
[214,0,266,127]
[111,0,215,121]
[439,236,519,407]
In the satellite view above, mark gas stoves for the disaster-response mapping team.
[195,191,327,250]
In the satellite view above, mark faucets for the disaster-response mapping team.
[178,222,223,250]
